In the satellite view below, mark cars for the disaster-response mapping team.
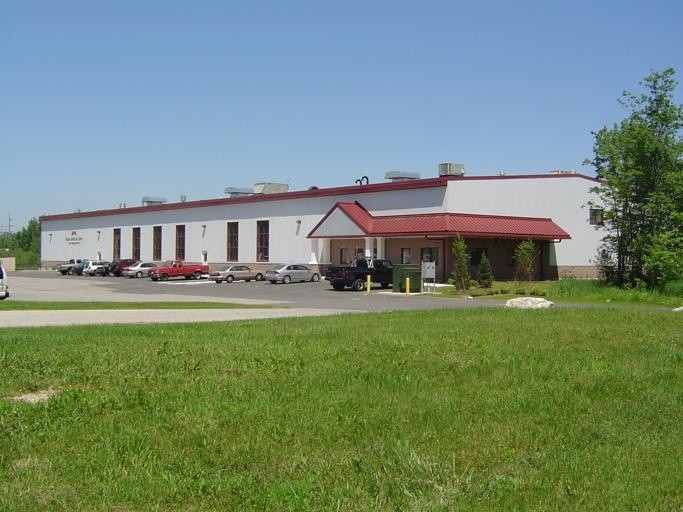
[59,258,320,284]
[0,260,9,299]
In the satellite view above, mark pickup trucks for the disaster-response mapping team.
[325,256,394,291]
